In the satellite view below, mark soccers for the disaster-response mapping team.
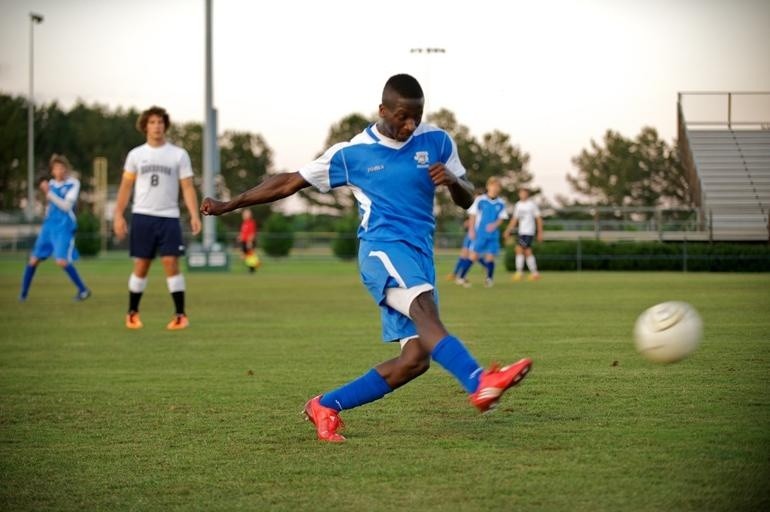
[635,301,702,364]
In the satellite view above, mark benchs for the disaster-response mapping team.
[680,129,770,239]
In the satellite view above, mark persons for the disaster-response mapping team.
[199,74,537,447]
[448,231,490,278]
[499,187,543,280]
[236,206,258,274]
[110,106,203,331]
[18,154,92,302]
[456,173,504,289]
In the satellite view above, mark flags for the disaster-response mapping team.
[31,10,45,24]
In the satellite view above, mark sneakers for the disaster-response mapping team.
[445,270,541,288]
[73,289,91,302]
[167,314,189,331]
[466,357,533,414]
[302,393,347,443]
[125,312,142,330]
